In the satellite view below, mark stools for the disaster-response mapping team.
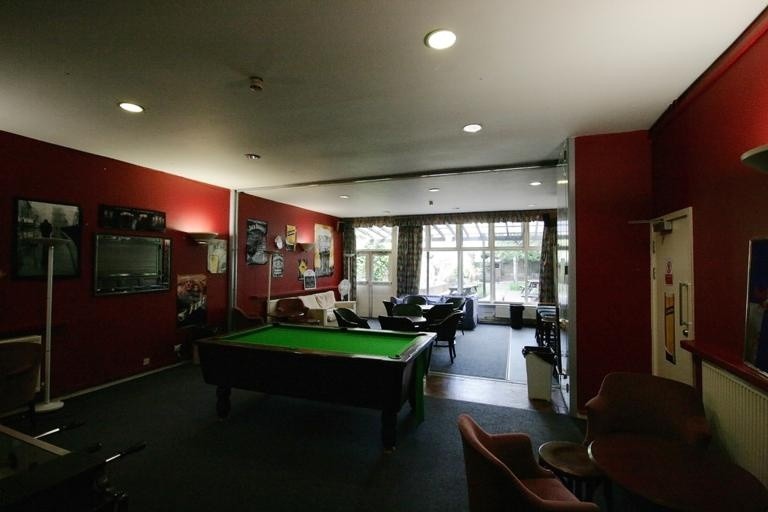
[538,439,601,502]
[534,303,556,350]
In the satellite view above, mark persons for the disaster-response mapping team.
[177,275,207,311]
[38,219,53,238]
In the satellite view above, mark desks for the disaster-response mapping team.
[526,280,539,295]
[448,283,479,297]
[192,321,439,454]
[588,434,768,511]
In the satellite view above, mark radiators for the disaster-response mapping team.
[700,359,768,490]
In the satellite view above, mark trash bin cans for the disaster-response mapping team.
[522,346,555,402]
[510,305,525,329]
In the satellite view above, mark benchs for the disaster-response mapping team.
[519,285,534,293]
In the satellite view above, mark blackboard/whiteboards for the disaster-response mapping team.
[304,275,316,290]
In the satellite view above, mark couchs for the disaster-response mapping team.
[298,290,356,326]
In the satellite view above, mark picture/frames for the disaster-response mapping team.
[9,197,83,282]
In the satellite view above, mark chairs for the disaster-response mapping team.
[581,370,706,435]
[273,299,310,326]
[455,413,602,512]
[332,292,479,364]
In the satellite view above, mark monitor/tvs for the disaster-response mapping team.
[93,233,172,298]
